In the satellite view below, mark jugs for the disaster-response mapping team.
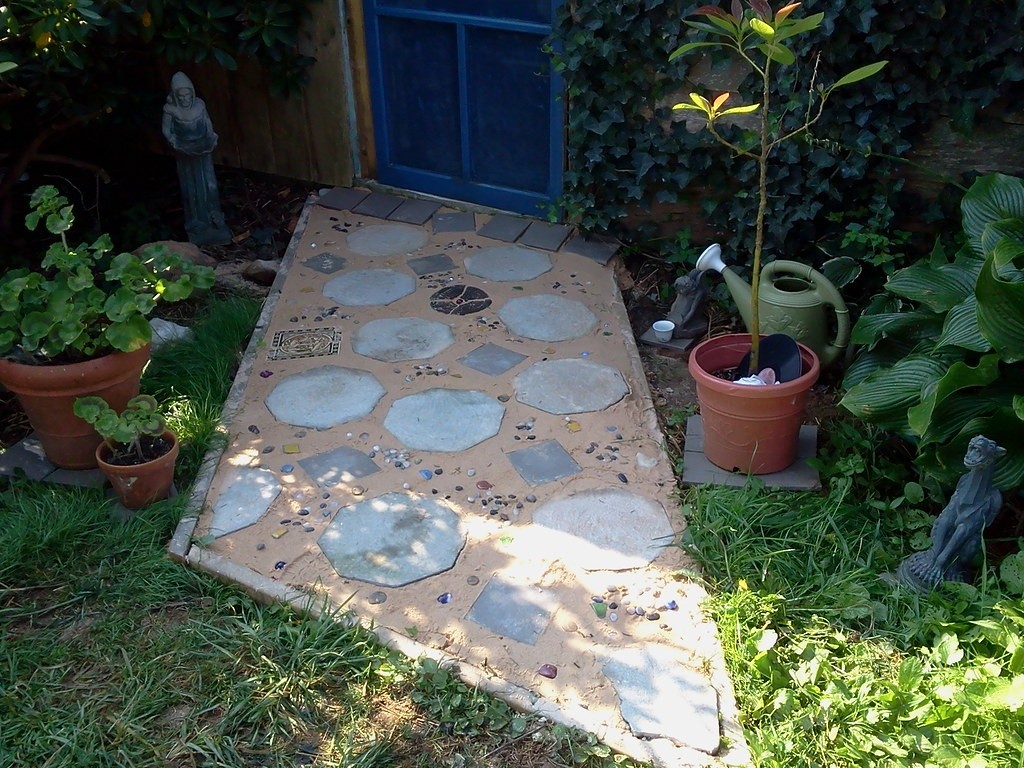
[696,242,851,371]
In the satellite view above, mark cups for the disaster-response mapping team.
[652,320,675,343]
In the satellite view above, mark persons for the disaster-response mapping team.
[160,71,225,233]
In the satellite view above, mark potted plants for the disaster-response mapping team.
[0,184,218,472]
[669,0,890,476]
[74,394,179,509]
